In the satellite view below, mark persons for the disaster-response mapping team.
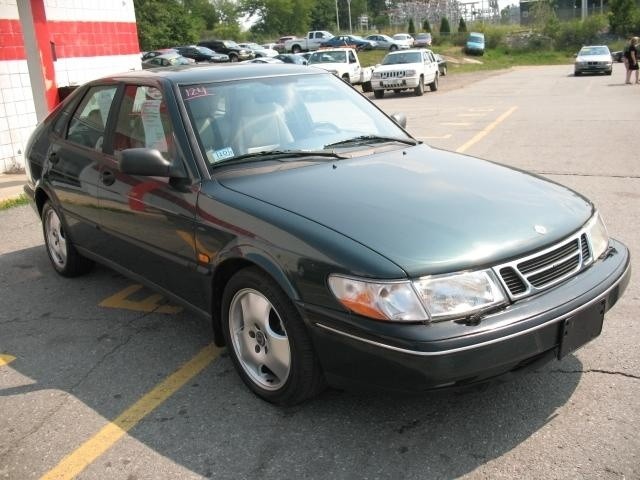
[625,36,640,84]
[623,37,632,77]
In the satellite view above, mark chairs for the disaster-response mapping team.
[69,84,294,155]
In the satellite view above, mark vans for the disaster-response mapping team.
[464,31,486,56]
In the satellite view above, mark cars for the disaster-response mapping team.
[572,43,617,76]
[23,62,630,409]
